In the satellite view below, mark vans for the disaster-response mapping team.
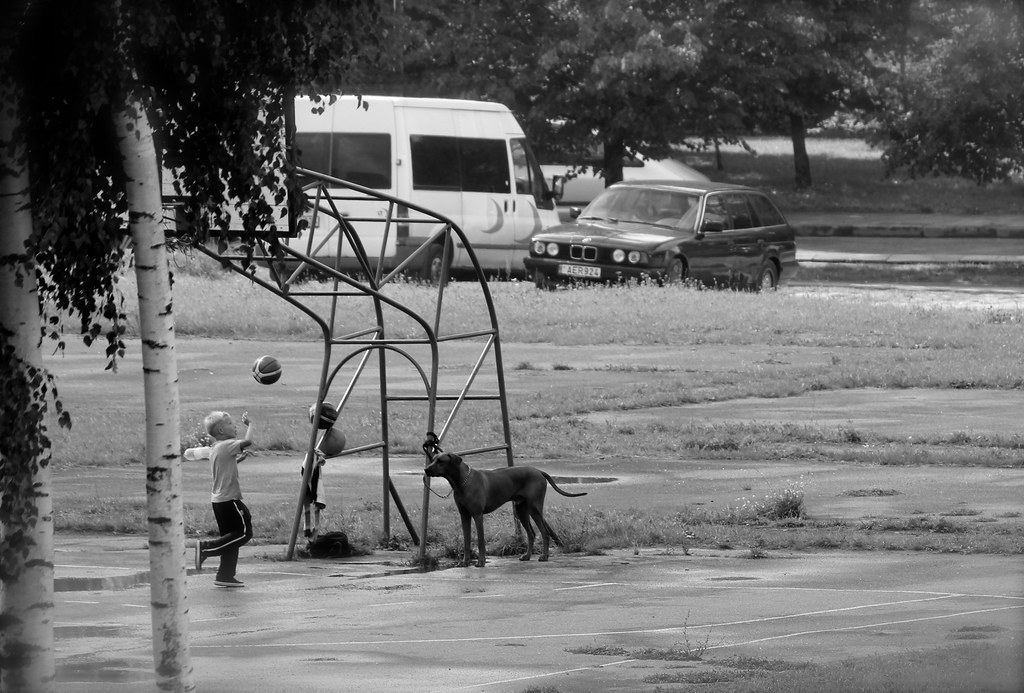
[202,95,563,283]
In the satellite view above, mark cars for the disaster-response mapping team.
[525,120,711,207]
[520,180,799,296]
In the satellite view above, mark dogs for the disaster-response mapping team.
[425,452,589,567]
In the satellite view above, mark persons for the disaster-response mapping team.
[195,411,253,586]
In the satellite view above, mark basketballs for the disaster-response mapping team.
[251,354,282,386]
[316,426,346,456]
[308,401,338,430]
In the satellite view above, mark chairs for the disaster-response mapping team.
[658,203,684,219]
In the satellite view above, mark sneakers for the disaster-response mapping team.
[195,540,207,571]
[213,573,244,586]
